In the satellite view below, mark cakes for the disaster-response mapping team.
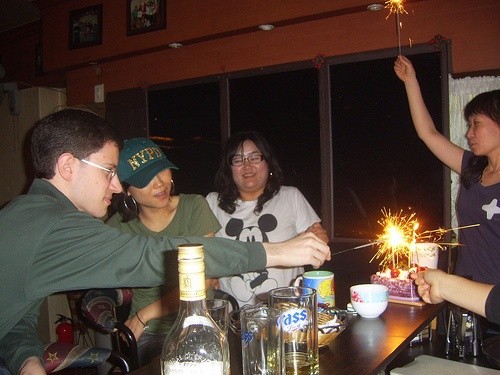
[370,260,431,301]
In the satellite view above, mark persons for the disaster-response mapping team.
[202,129,328,314]
[394,55,500,330]
[410,269,500,328]
[104,136,223,375]
[0,106,331,375]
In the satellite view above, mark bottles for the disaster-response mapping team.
[161,244,230,375]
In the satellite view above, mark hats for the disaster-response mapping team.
[118,138,179,188]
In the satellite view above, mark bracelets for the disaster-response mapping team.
[135,312,148,326]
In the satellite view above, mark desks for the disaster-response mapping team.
[128,242,458,375]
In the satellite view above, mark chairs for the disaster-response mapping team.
[80,286,240,370]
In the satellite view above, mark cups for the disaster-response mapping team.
[203,300,229,342]
[293,270,336,314]
[239,308,269,375]
[414,242,438,269]
[267,286,320,375]
[350,284,389,318]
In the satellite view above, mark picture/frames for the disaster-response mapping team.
[68,4,102,50]
[126,0,167,36]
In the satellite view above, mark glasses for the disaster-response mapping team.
[74,157,117,180]
[231,155,265,165]
